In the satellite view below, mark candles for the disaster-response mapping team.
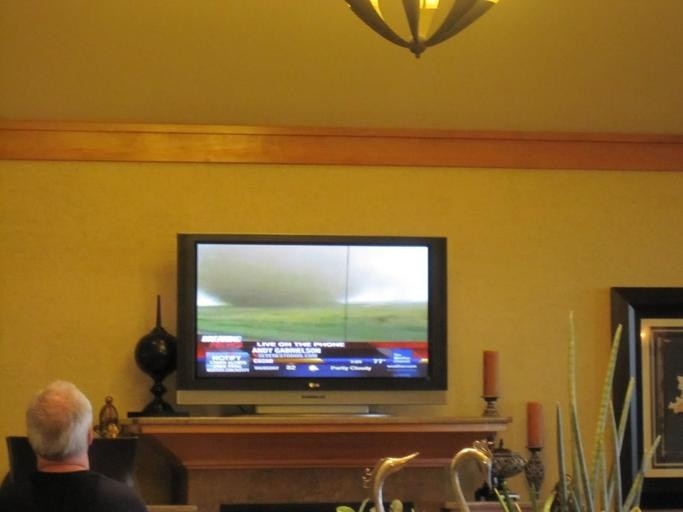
[482,350,500,396]
[527,401,543,449]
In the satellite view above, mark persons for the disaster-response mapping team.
[0,381,149,512]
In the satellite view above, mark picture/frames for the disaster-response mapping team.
[608,281,683,511]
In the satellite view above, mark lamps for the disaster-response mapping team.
[345,0,496,59]
